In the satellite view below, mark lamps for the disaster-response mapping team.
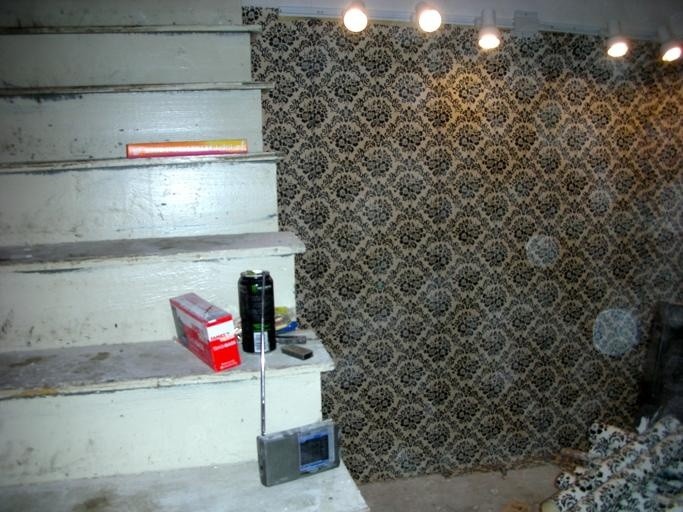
[417,2,443,34]
[656,24,682,62]
[342,3,370,33]
[605,18,629,58]
[478,7,502,52]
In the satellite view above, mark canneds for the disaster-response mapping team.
[238,269,276,354]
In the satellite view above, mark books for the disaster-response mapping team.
[126,140,248,158]
